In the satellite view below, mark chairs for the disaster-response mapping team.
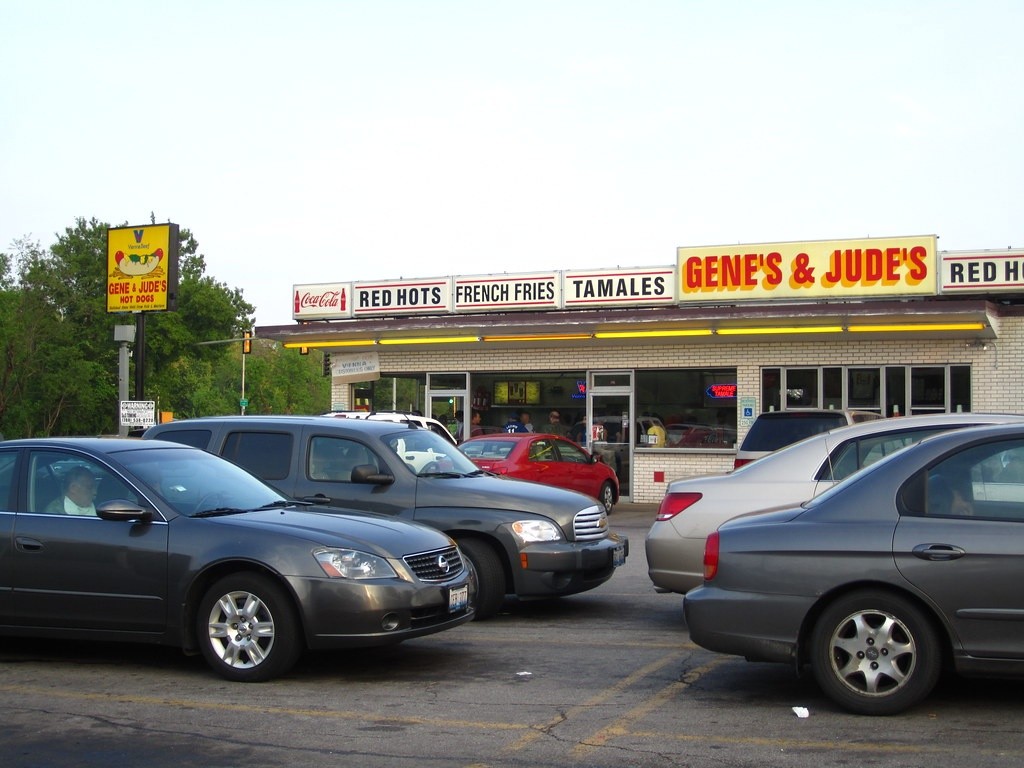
[496,448,511,458]
[93,476,129,500]
[35,480,62,514]
[465,448,481,458]
[312,455,330,479]
[929,474,951,513]
[483,452,496,457]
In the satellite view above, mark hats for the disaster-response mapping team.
[454,411,463,418]
[508,413,519,419]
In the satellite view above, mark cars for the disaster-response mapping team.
[682,421,1024,715]
[437,431,620,516]
[0,434,481,685]
[566,413,737,449]
[642,411,1024,598]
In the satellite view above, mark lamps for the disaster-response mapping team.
[483,333,593,342]
[593,327,716,338]
[846,322,991,332]
[282,339,377,348]
[449,397,453,403]
[716,325,846,335]
[378,335,481,345]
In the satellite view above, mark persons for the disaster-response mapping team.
[647,419,666,447]
[541,411,569,438]
[503,412,530,433]
[410,409,463,445]
[460,410,484,441]
[616,409,643,444]
[47,465,97,517]
[519,413,536,433]
[577,416,620,447]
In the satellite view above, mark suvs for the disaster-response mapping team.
[732,408,909,472]
[315,409,456,478]
[137,414,627,621]
